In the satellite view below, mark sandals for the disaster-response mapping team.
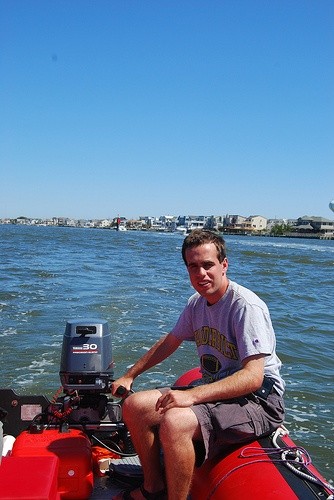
[113,484,162,500]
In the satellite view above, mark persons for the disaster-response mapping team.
[110,230,285,500]
